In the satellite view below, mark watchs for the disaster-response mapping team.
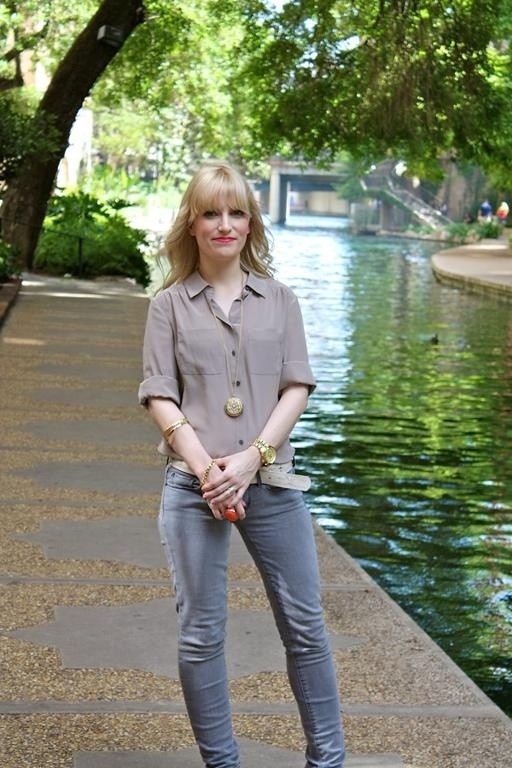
[251,439,279,466]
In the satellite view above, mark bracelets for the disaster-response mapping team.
[163,416,188,440]
[191,458,218,492]
[159,417,190,435]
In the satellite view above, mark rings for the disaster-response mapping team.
[223,504,239,521]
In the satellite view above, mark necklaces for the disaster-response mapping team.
[193,263,246,420]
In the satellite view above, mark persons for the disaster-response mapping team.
[495,197,510,226]
[463,209,474,224]
[477,196,492,223]
[138,164,345,767]
[440,201,447,215]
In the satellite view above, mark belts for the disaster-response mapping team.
[168,459,312,492]
